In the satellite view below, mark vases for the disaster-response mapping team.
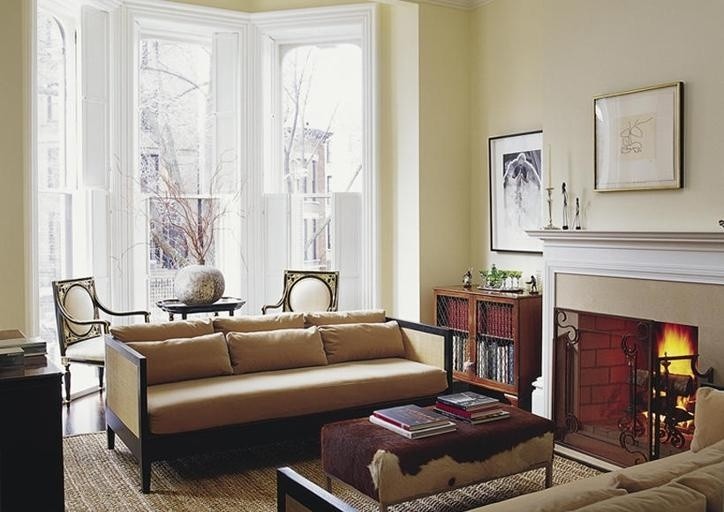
[174,264,226,307]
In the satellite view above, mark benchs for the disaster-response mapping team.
[320,402,555,512]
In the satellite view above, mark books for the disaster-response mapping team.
[433,391,511,424]
[0,336,50,372]
[367,403,457,441]
[437,297,515,385]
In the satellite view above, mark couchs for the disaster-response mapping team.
[102,311,453,495]
[275,382,724,511]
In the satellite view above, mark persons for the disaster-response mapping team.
[511,154,529,204]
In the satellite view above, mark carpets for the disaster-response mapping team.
[63,428,609,512]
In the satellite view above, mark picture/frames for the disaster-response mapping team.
[592,80,686,195]
[486,129,543,255]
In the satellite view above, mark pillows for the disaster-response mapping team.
[123,319,405,386]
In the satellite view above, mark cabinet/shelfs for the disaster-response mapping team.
[0,357,70,512]
[430,285,542,412]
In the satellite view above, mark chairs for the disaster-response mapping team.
[51,276,151,407]
[261,269,339,315]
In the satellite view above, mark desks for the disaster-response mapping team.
[155,296,246,322]
